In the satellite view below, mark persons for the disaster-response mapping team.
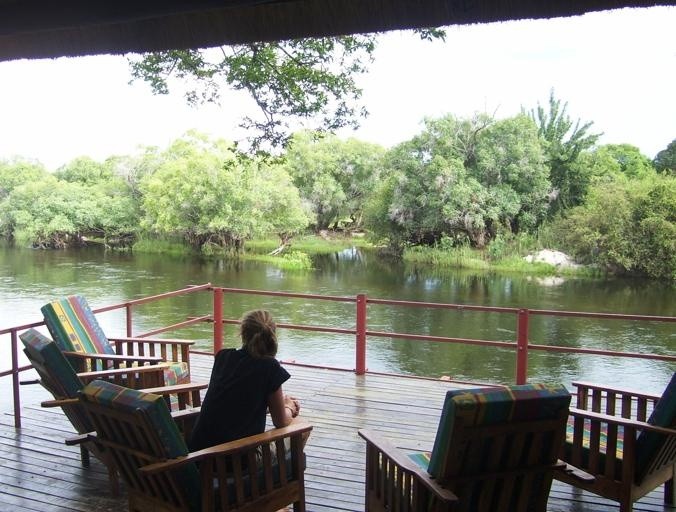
[189,308,300,475]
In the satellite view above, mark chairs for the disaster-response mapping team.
[62,337,195,404]
[20,364,210,497]
[357,429,595,512]
[66,407,312,512]
[568,382,676,512]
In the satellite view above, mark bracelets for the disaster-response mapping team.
[284,405,295,414]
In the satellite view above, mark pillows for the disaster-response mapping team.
[82,379,204,495]
[18,327,92,426]
[428,383,572,512]
[633,373,676,480]
[40,295,117,371]
